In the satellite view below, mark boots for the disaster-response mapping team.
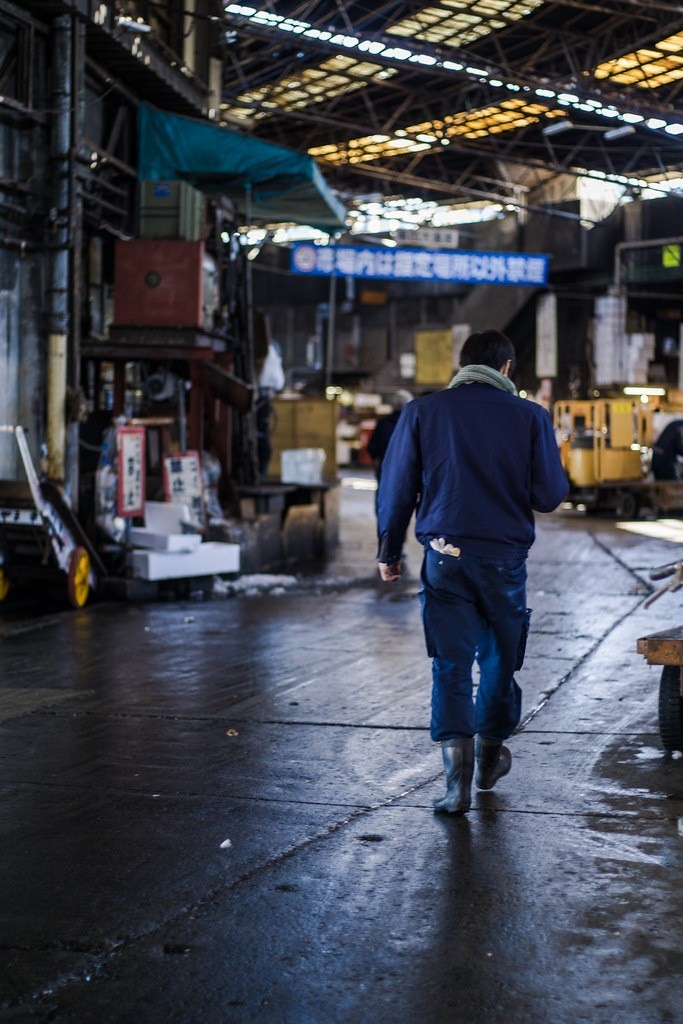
[475,733,512,790]
[433,738,475,812]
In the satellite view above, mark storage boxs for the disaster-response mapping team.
[131,501,241,585]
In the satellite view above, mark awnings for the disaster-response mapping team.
[139,101,349,226]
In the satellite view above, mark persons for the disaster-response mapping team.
[373,328,570,815]
[367,386,418,570]
[649,421,683,519]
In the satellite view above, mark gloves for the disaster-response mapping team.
[430,537,460,557]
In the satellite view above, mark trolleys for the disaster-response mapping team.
[0,424,112,610]
